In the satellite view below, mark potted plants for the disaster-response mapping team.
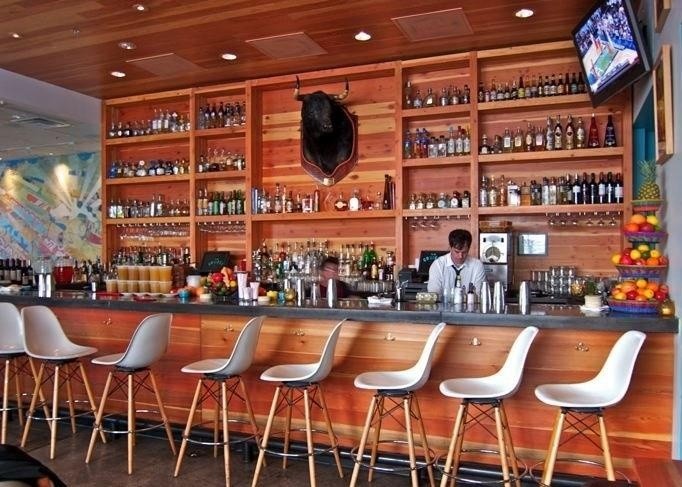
[651,44,673,166]
[517,232,549,257]
[654,0,669,29]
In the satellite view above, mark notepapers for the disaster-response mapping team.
[636,157,661,201]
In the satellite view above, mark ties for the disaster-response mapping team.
[569,0,652,109]
[200,250,230,274]
[417,251,450,274]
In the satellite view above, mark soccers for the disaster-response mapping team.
[654,292,665,300]
[626,290,638,300]
[638,222,653,232]
[658,284,668,297]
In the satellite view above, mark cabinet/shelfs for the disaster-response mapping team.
[108,102,245,267]
[453,266,616,315]
[92,293,96,304]
[404,71,623,210]
[0,256,101,298]
[252,174,393,309]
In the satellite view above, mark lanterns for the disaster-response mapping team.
[636,279,648,289]
[630,250,641,260]
[650,248,660,258]
[637,244,649,253]
[611,254,621,263]
[613,292,626,301]
[644,288,654,300]
[646,214,659,226]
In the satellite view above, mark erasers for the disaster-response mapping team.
[291,74,353,175]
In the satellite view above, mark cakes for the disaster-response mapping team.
[522,265,593,296]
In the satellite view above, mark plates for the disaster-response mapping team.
[100,40,633,300]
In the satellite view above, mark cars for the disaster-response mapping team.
[180,298,189,304]
[237,273,259,302]
[106,266,173,294]
[180,275,201,298]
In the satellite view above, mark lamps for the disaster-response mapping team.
[349,321,447,487]
[0,300,57,445]
[250,320,346,486]
[20,305,107,459]
[85,314,176,473]
[172,316,269,486]
[438,326,541,487]
[534,329,648,487]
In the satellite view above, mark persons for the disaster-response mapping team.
[427,229,487,304]
[317,256,350,299]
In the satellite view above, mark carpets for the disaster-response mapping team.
[452,265,464,287]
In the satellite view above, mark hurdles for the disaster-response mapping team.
[620,281,636,295]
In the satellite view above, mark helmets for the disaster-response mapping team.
[119,292,179,298]
